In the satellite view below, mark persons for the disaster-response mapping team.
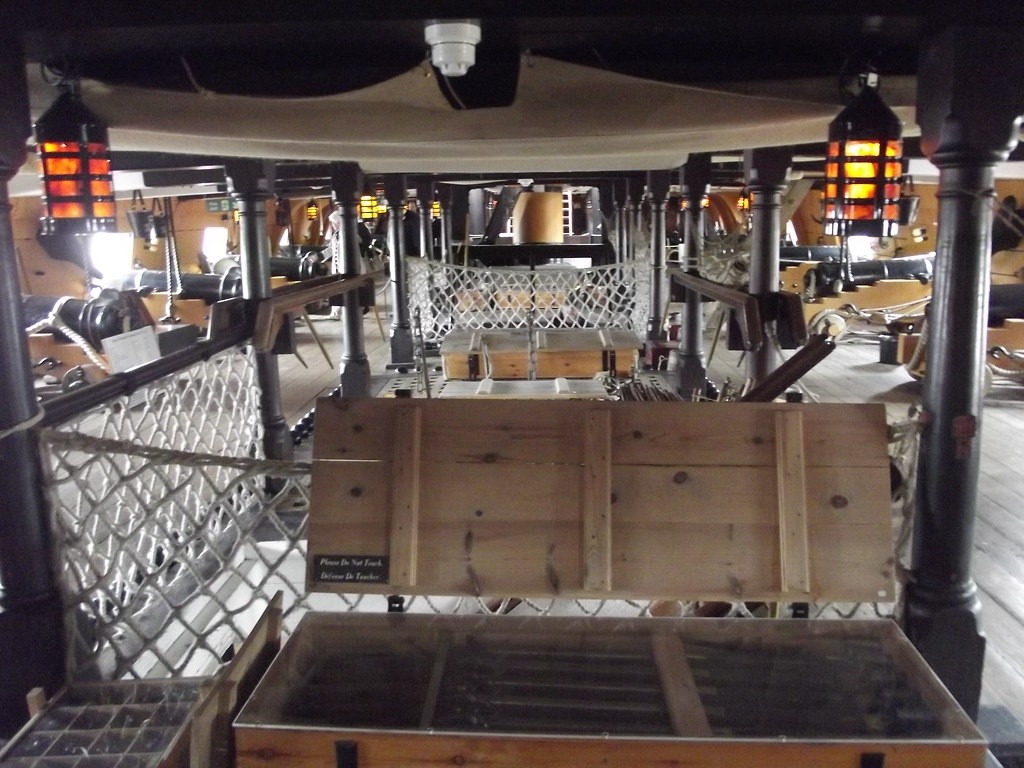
[433,216,441,246]
[309,211,344,321]
[358,217,374,316]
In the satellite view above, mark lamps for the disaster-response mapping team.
[432,198,442,218]
[821,84,903,239]
[33,91,119,237]
[680,198,691,209]
[305,198,320,223]
[233,208,241,225]
[358,183,379,220]
[376,189,387,215]
[700,195,711,209]
[736,189,752,211]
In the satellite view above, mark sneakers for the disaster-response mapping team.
[323,305,342,322]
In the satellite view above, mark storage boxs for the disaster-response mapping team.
[229,387,992,767]
[0,588,287,767]
[440,326,646,381]
